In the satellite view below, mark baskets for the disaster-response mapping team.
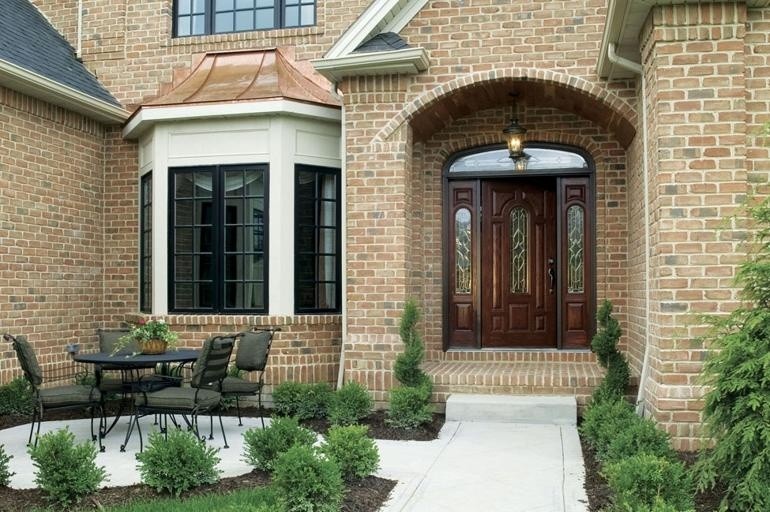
[136,338,167,354]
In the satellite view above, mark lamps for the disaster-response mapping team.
[513,153,531,171]
[500,90,527,163]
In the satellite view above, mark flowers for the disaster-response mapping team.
[108,313,178,359]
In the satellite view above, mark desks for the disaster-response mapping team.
[73,347,209,439]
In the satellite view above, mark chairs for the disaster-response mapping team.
[97,327,283,453]
[2,332,106,453]
